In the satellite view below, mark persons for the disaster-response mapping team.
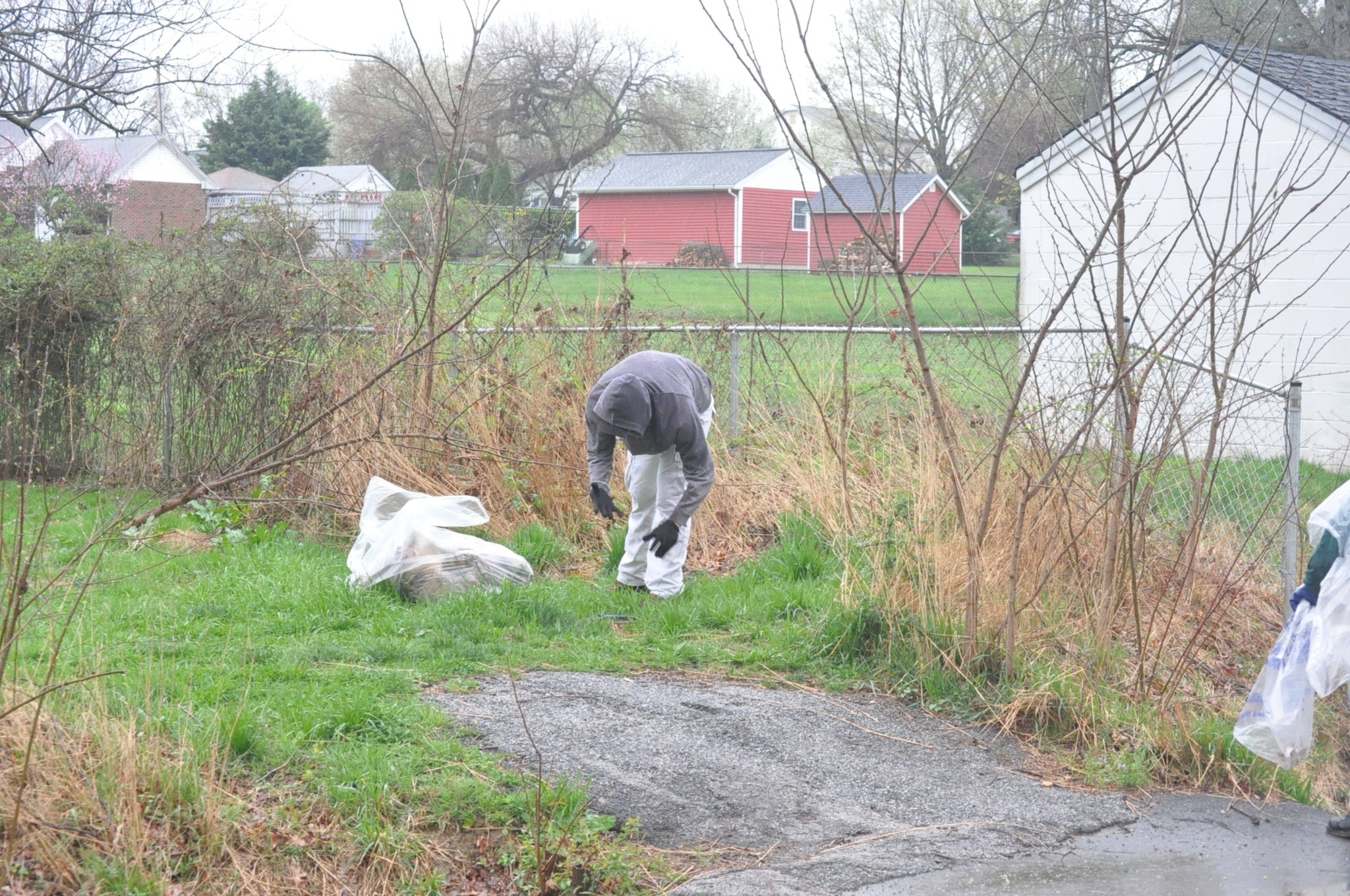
[584,350,716,600]
[1289,475,1350,839]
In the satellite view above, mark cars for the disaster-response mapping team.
[1007,230,1021,253]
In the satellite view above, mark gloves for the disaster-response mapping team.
[642,520,681,558]
[590,481,624,520]
[1289,584,1319,612]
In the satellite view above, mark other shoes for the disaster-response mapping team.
[1326,812,1350,838]
